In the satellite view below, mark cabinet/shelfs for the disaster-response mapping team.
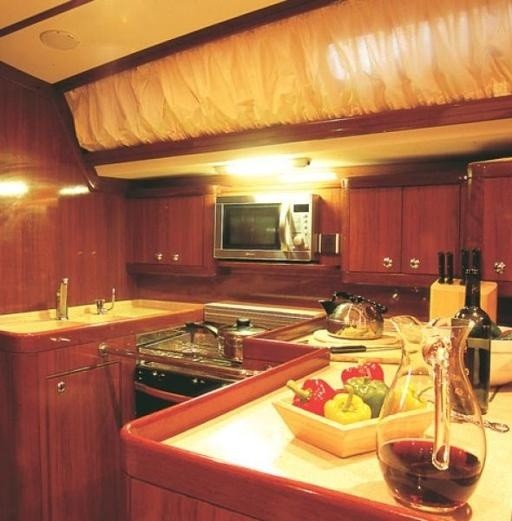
[128,185,233,277]
[0,332,136,521]
[341,159,512,296]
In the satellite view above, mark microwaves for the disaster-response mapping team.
[213,193,317,263]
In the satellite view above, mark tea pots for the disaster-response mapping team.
[318,290,388,339]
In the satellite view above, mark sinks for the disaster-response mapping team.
[32,318,87,333]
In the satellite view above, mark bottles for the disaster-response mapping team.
[375,314,487,468]
[450,267,492,416]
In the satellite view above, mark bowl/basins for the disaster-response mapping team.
[272,392,434,458]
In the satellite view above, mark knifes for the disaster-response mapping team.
[435,248,482,285]
[329,344,401,354]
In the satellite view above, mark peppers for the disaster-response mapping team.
[286,359,433,442]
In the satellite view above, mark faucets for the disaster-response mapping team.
[95,286,116,315]
[56,277,70,318]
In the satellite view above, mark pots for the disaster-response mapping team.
[184,319,269,363]
